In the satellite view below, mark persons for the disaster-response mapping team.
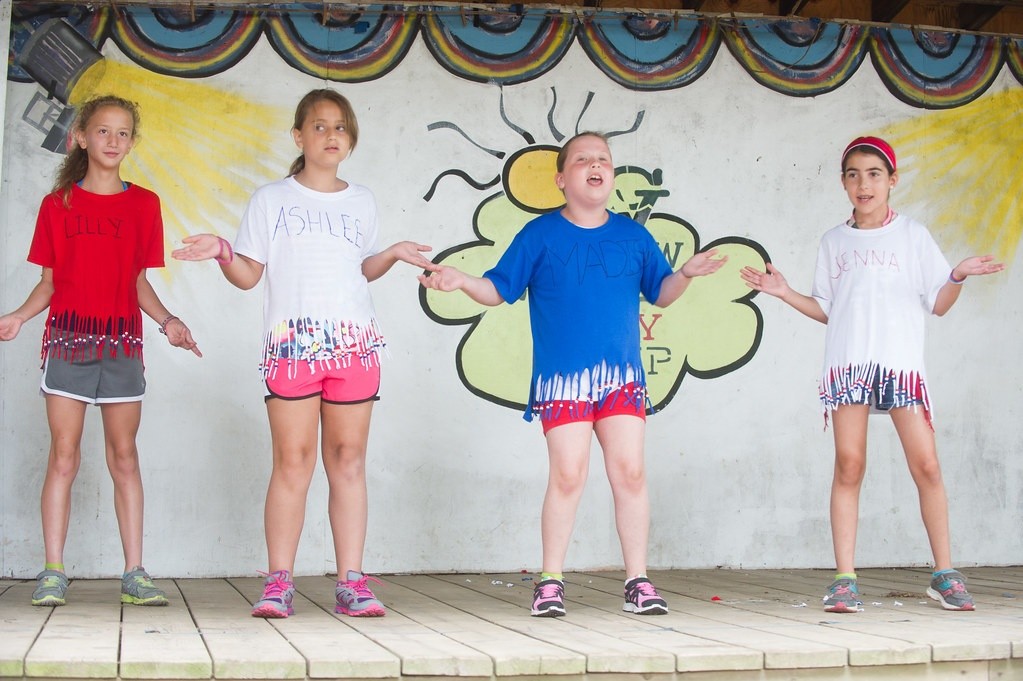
[417,131,728,618]
[740,136,1004,612]
[172,89,443,618]
[0,95,202,607]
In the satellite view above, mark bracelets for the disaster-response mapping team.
[682,263,692,278]
[949,269,968,284]
[216,236,233,265]
[158,315,179,335]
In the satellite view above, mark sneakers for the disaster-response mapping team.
[531,579,567,616]
[822,579,860,613]
[252,569,295,618]
[622,574,670,615]
[120,566,170,606]
[925,570,977,611]
[32,569,68,605]
[333,570,386,617]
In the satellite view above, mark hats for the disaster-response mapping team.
[842,136,897,174]
